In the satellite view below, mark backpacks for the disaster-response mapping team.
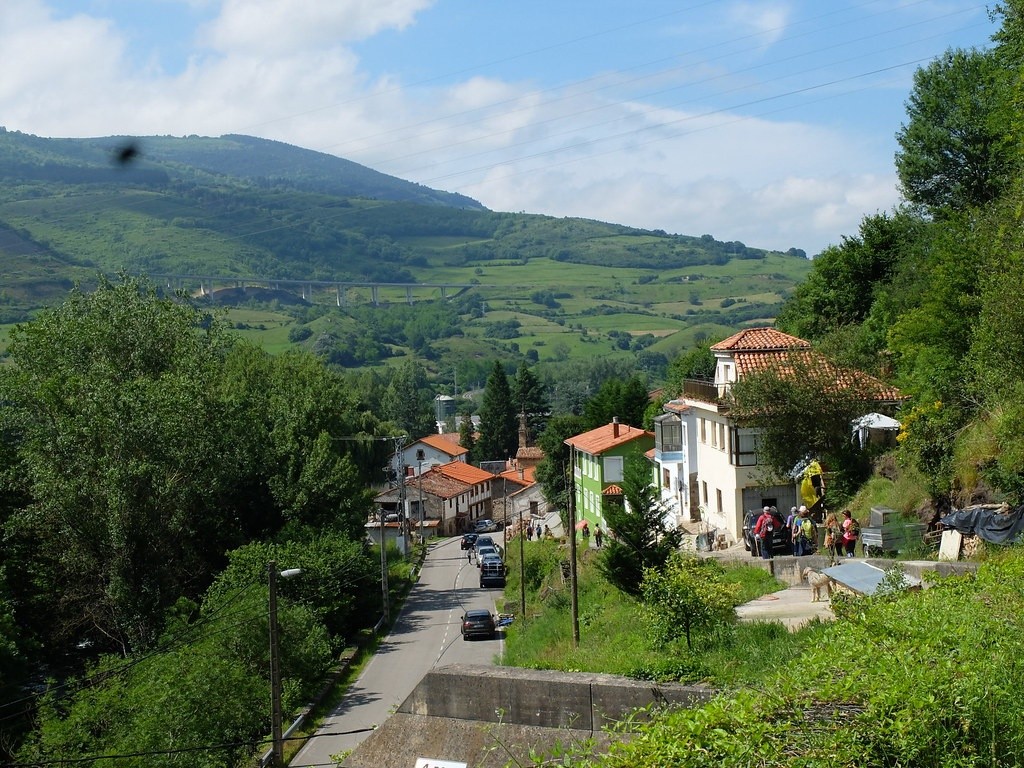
[801,519,813,539]
[848,518,860,536]
[764,514,774,540]
[832,523,843,543]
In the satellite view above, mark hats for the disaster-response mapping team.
[791,506,796,511]
[763,506,770,513]
[799,506,809,512]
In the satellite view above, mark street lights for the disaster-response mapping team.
[267,567,303,768]
[380,513,400,625]
[417,461,430,544]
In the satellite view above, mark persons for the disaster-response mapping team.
[495,544,503,559]
[583,523,604,547]
[466,545,474,565]
[525,523,549,541]
[754,506,858,568]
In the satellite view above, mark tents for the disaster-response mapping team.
[853,412,903,451]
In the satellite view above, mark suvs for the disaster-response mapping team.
[742,505,793,557]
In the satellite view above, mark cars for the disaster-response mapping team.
[472,535,507,589]
[460,534,479,550]
[460,610,495,640]
[474,520,499,533]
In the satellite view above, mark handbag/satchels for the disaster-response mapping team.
[841,536,849,547]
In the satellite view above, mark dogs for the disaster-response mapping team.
[803,562,841,602]
